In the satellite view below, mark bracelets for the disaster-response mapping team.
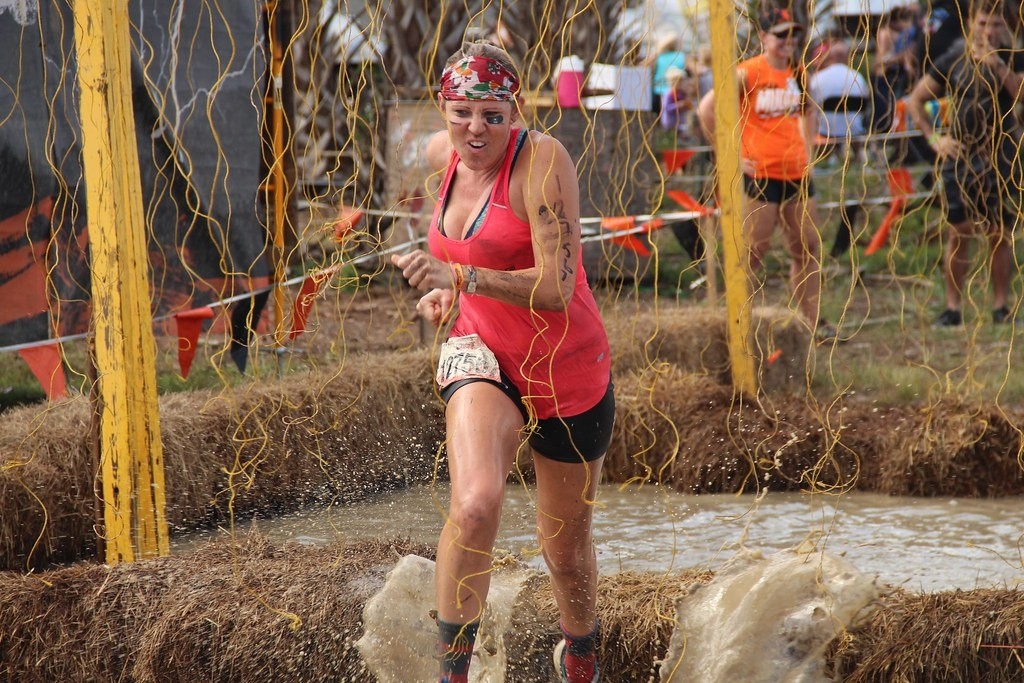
[995,60,1005,72]
[928,133,941,143]
[449,261,477,294]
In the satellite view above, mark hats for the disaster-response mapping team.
[757,9,797,32]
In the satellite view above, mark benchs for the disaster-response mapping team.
[812,126,948,255]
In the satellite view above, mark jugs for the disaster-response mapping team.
[558,53,584,107]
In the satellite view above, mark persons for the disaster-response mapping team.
[697,7,850,345]
[387,40,615,683]
[908,0,1024,324]
[806,40,872,164]
[873,7,962,144]
[618,27,713,160]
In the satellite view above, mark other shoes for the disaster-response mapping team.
[992,306,1012,323]
[553,639,599,683]
[811,319,848,345]
[930,308,961,332]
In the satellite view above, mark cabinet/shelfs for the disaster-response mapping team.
[380,98,657,282]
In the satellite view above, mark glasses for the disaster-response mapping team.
[766,25,802,39]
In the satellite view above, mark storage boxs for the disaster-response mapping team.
[587,64,652,112]
